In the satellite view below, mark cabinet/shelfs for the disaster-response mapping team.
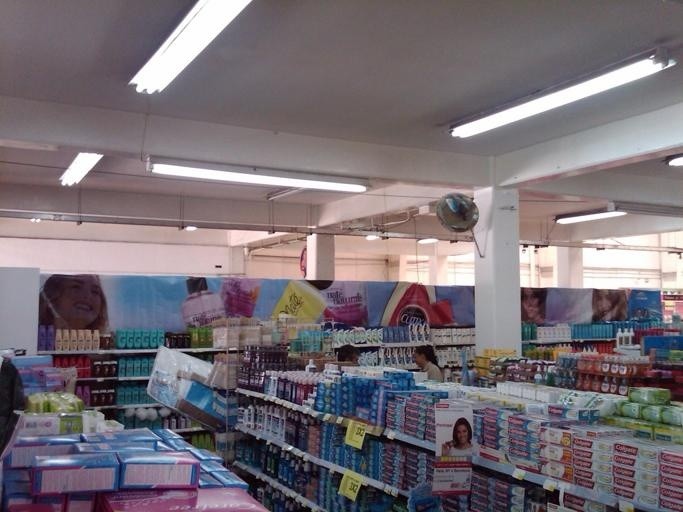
[1,318,681,512]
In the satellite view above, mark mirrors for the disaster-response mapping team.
[435,192,481,235]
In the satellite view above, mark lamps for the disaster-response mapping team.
[549,206,629,228]
[141,153,371,195]
[56,151,104,187]
[665,146,683,172]
[444,37,678,140]
[125,1,256,95]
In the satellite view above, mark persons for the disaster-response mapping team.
[337,344,363,366]
[39,273,109,331]
[591,289,628,322]
[438,416,476,457]
[412,344,444,384]
[520,287,546,324]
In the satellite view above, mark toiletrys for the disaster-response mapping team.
[39,319,683,511]
